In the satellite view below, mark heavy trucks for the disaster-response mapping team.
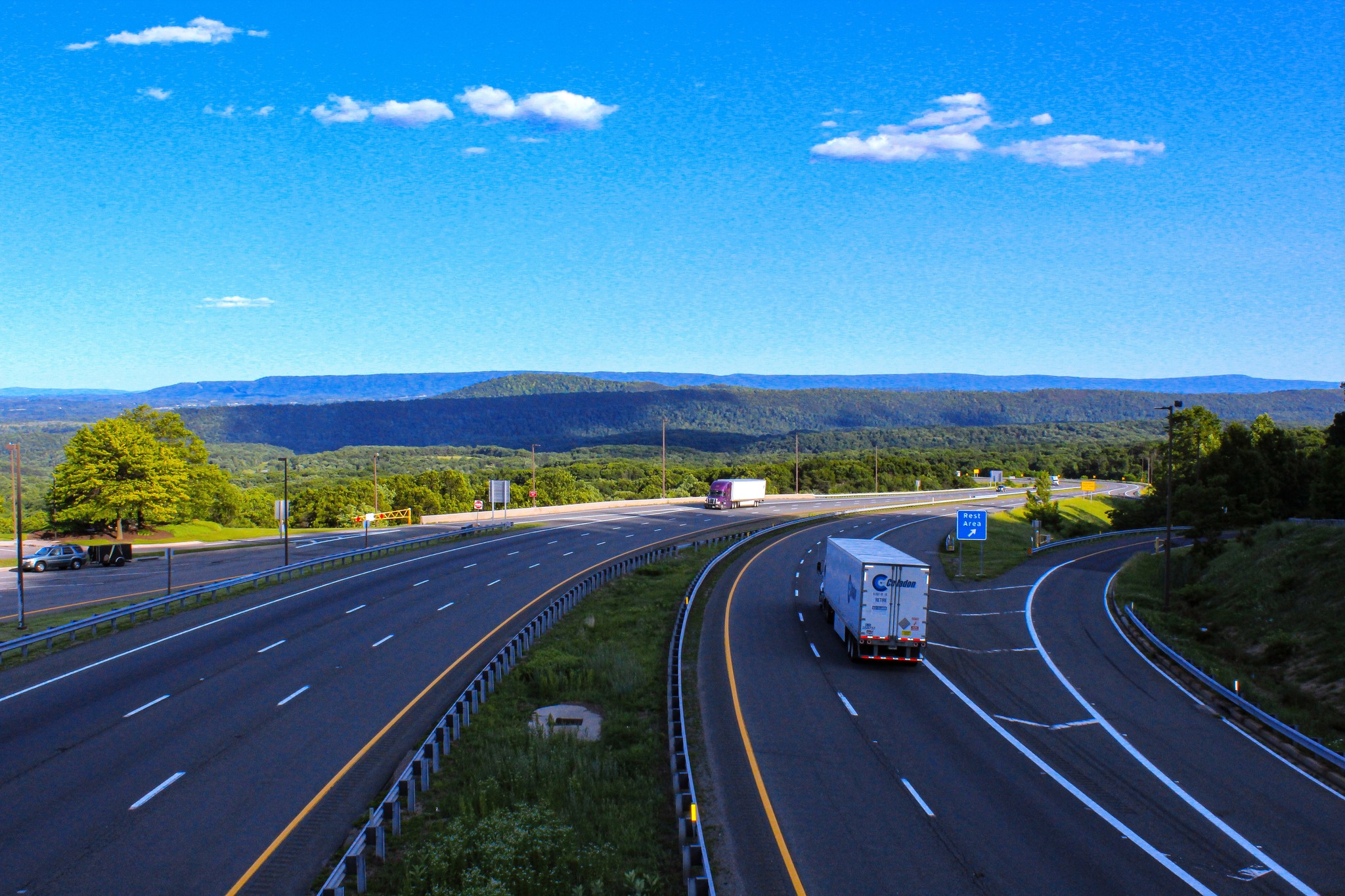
[816,537,933,668]
[704,479,767,511]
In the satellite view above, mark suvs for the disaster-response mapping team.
[23,544,86,573]
[996,485,1006,492]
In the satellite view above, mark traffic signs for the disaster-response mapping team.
[956,510,989,542]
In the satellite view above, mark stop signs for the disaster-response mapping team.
[475,501,482,509]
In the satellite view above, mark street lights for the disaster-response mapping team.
[531,443,542,509]
[277,457,288,567]
[875,444,878,492]
[661,419,669,500]
[1153,406,1172,615]
[374,452,379,526]
[795,433,798,493]
[1189,430,1201,530]
[5,443,26,631]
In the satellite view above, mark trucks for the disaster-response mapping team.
[1051,476,1060,486]
